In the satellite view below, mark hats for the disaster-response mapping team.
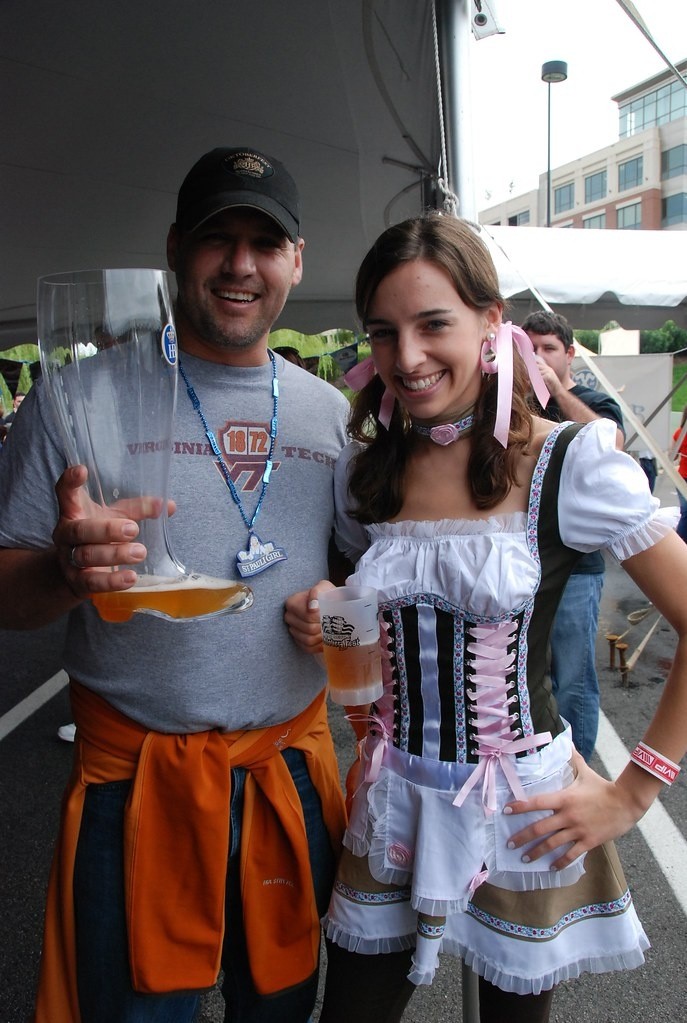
[176,147,302,244]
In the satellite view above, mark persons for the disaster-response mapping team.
[0,144,361,1023]
[516,312,623,763]
[283,212,687,1023]
[0,344,687,544]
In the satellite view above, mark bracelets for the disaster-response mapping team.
[629,741,681,789]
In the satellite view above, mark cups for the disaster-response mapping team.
[36,266,254,624]
[317,585,384,707]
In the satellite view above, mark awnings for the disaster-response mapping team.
[471,222,687,333]
[1,1,462,460]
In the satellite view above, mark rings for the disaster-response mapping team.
[72,546,85,569]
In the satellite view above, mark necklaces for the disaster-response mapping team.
[176,348,287,577]
[411,413,476,447]
